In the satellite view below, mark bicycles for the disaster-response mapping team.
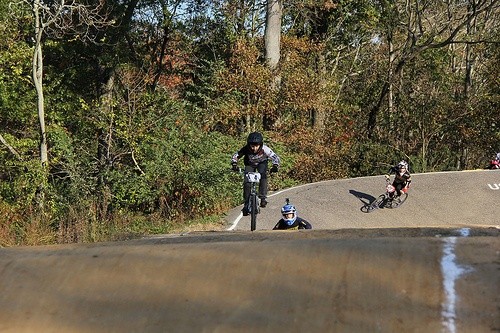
[367,174,409,213]
[231,166,279,231]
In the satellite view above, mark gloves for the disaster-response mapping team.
[270,164,279,173]
[232,162,238,171]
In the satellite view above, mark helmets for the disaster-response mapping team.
[281,204,297,226]
[247,131,263,155]
[398,160,408,176]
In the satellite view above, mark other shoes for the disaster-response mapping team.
[261,199,268,207]
[243,206,250,216]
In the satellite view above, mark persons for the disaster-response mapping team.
[385,160,411,200]
[232,132,281,216]
[272,204,312,230]
